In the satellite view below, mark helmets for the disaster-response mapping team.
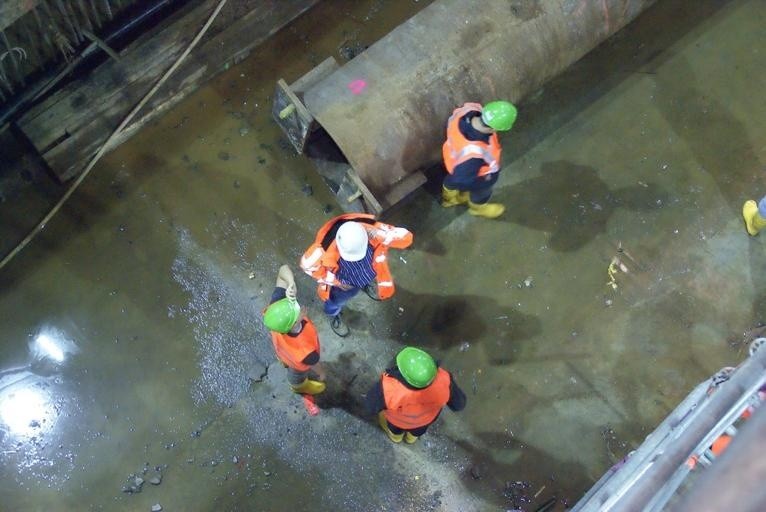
[335,220,369,262]
[263,297,301,335]
[481,100,517,132]
[395,346,438,389]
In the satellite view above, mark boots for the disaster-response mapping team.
[440,183,470,209]
[466,196,506,219]
[741,199,766,238]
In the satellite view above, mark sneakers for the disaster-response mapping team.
[378,409,405,443]
[404,431,419,444]
[288,375,327,396]
[328,312,350,338]
[364,284,380,302]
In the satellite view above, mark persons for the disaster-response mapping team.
[300,214,415,338]
[742,195,766,236]
[261,263,327,397]
[363,342,467,447]
[437,98,518,220]
